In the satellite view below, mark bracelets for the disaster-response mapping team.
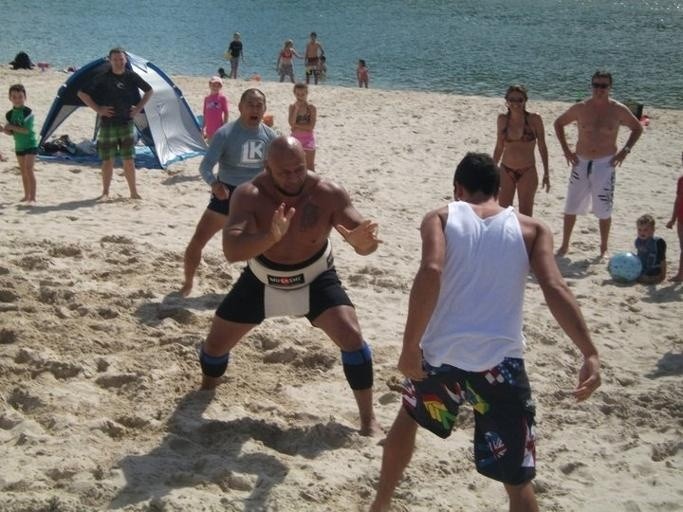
[544,176,549,178]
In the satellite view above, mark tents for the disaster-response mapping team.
[36,51,209,170]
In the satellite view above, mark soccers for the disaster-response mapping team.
[609,251,642,283]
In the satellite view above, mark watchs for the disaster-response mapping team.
[623,145,630,153]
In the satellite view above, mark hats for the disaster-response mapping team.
[211,76,223,87]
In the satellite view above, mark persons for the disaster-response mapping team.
[218,31,369,89]
[492,86,552,215]
[666,152,683,282]
[201,77,229,143]
[289,83,315,173]
[635,215,666,284]
[199,135,387,437]
[0,84,39,207]
[177,88,277,298]
[77,47,153,204]
[370,151,602,512]
[553,70,642,257]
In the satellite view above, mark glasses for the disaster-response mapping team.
[592,82,609,89]
